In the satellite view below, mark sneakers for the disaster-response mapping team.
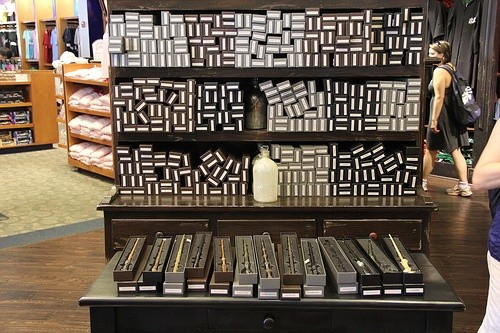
[446,185,472,197]
[422,179,428,192]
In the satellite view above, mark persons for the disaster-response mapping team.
[422,40,473,196]
[0,47,12,69]
[472,118,500,333]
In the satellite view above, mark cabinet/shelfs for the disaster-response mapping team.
[0,0,465,333]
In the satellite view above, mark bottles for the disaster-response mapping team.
[252,145,278,204]
[245,79,267,130]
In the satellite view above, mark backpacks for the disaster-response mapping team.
[438,64,481,126]
